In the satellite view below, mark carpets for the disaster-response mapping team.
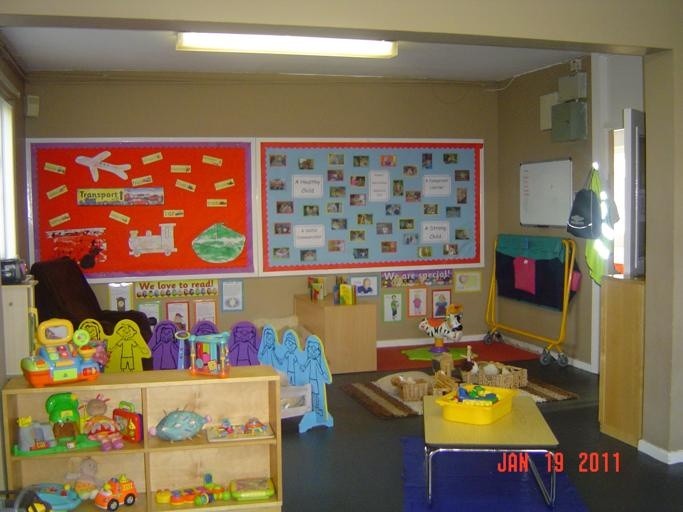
[377,340,540,370]
[339,365,580,419]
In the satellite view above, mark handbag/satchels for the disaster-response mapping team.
[566,168,602,239]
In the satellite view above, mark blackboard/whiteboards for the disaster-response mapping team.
[492,242,566,313]
[518,157,575,229]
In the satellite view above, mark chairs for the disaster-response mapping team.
[29,257,154,370]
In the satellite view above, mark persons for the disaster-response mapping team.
[436,294,447,316]
[390,295,400,321]
[357,278,373,294]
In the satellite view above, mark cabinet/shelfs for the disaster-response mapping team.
[1,365,283,512]
[294,292,377,374]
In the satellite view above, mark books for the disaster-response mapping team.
[332,274,357,305]
[307,275,328,303]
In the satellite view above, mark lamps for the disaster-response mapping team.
[176,32,398,60]
